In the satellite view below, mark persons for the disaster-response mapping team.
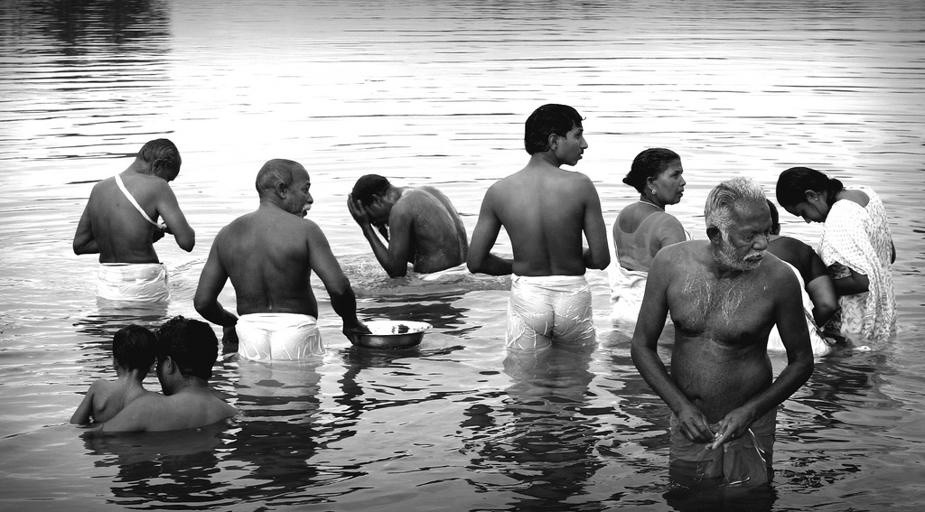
[606,146,695,323]
[764,198,838,352]
[71,138,196,302]
[69,323,156,424]
[345,172,471,281]
[466,102,611,351]
[191,157,373,360]
[630,174,814,483]
[102,314,239,435]
[775,165,899,348]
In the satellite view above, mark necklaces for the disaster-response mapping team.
[639,200,665,210]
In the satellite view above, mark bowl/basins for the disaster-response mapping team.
[361,318,433,349]
